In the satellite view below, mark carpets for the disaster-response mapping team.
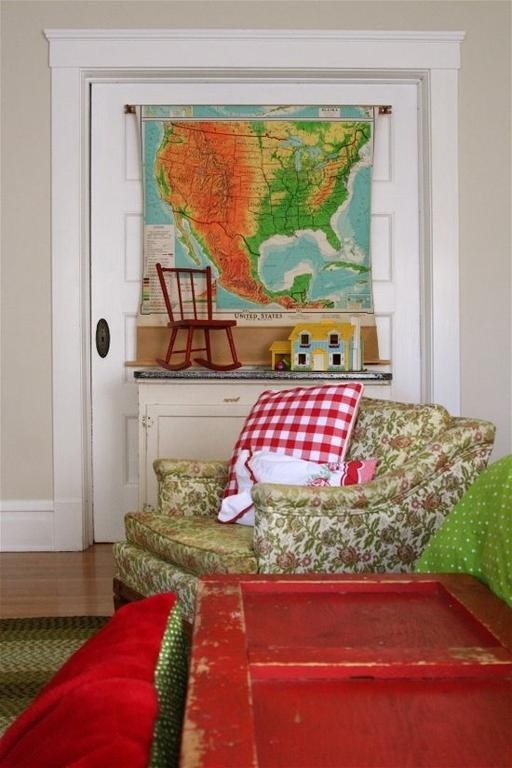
[0,615,122,741]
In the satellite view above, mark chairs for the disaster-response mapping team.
[155,261,242,372]
[110,399,496,622]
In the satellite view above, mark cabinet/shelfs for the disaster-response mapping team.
[130,366,397,521]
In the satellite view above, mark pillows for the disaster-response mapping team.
[214,383,380,531]
